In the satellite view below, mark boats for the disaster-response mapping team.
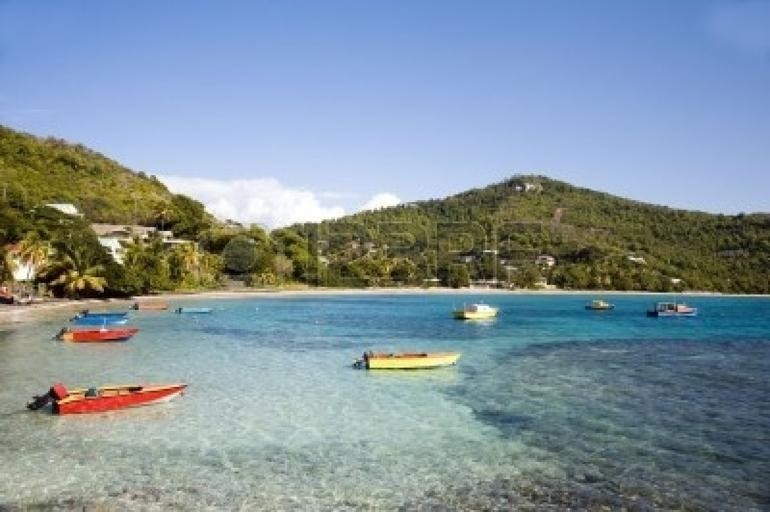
[174,306,211,314]
[585,300,615,311]
[647,302,697,318]
[453,303,498,320]
[69,311,128,327]
[353,351,458,370]
[135,303,168,310]
[56,328,139,343]
[27,384,186,416]
